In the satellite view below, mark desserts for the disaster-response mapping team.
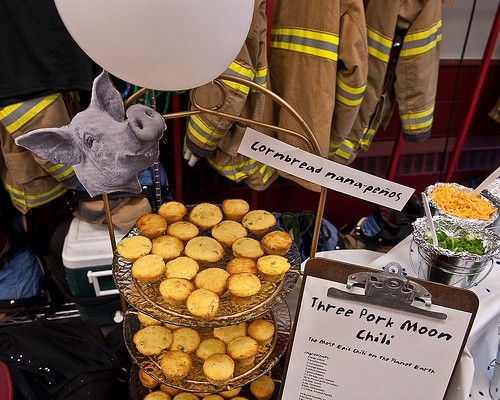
[118,199,292,400]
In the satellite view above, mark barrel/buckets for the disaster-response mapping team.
[409,231,495,290]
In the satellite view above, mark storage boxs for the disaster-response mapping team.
[62,218,126,328]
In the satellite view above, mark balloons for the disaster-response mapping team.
[55,0,255,91]
